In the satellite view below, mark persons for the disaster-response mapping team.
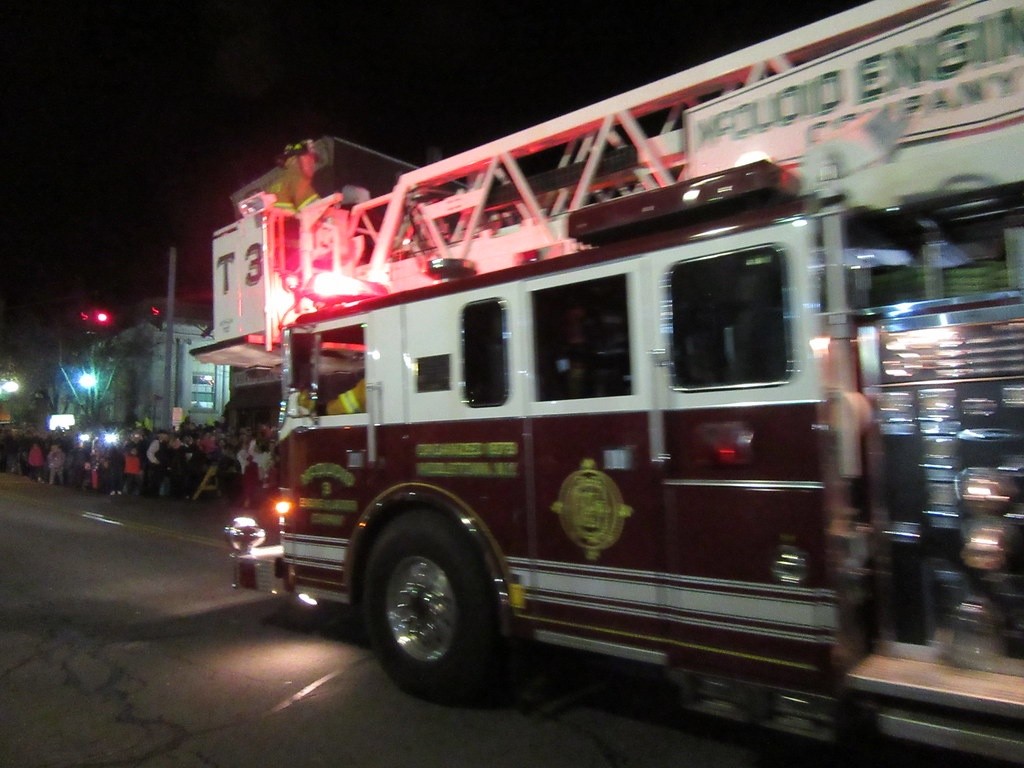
[1,417,281,510]
[550,304,602,399]
[327,377,366,416]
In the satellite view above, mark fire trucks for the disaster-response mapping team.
[192,0,1024,767]
